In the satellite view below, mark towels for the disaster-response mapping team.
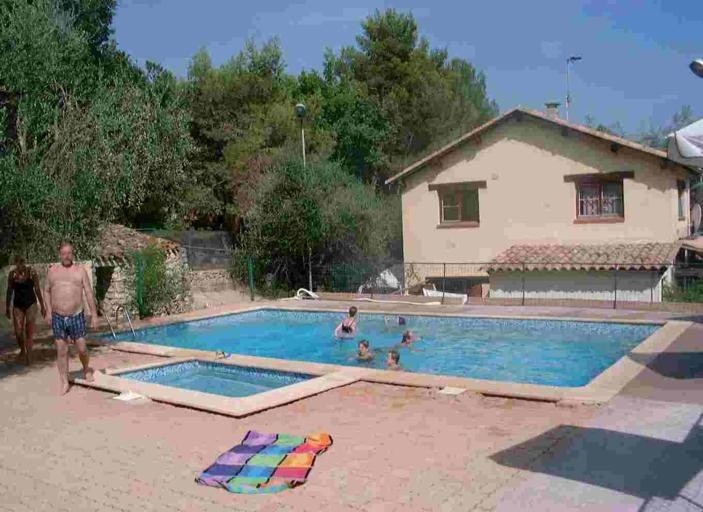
[191,429,335,494]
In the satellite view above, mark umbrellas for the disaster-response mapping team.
[663,116,702,170]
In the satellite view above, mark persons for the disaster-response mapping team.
[373,328,425,355]
[3,253,46,367]
[382,313,405,329]
[346,337,373,362]
[333,305,359,341]
[382,351,401,371]
[42,238,99,398]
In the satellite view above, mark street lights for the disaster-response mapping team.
[566,54,582,122]
[295,103,319,299]
